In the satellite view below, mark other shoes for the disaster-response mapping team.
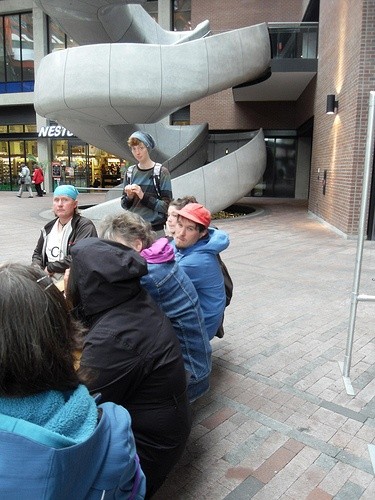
[28,196,33,198]
[16,195,21,198]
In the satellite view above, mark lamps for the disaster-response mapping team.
[326,94,338,116]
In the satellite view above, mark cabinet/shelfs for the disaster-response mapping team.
[1,152,121,186]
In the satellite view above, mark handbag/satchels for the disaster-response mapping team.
[16,176,25,185]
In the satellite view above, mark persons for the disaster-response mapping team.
[163,195,234,338]
[0,265,147,500]
[15,163,33,198]
[61,239,191,500]
[32,164,44,197]
[170,202,230,340]
[96,210,214,402]
[120,130,172,240]
[31,184,98,295]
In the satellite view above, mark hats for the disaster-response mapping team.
[176,202,212,229]
[53,184,80,201]
[130,130,156,152]
